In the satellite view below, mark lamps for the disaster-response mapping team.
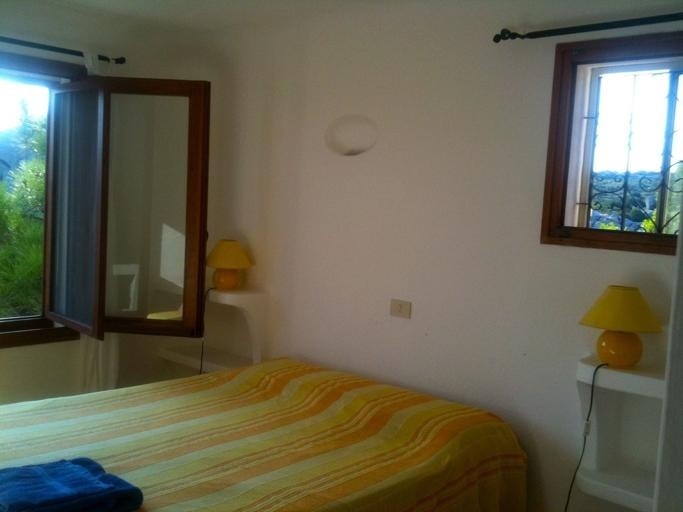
[577,284,664,369]
[204,239,251,291]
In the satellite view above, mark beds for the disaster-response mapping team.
[1,357,527,512]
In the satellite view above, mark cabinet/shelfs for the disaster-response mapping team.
[569,361,665,511]
[146,278,268,383]
